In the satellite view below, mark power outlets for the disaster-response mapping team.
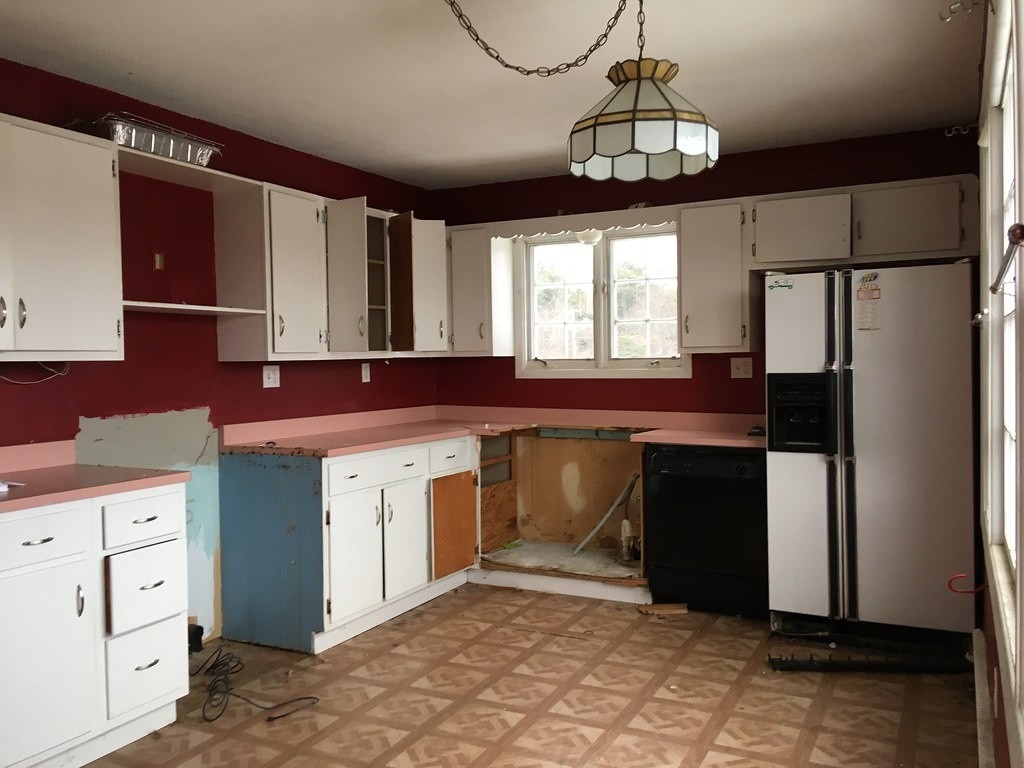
[731,357,753,379]
[361,363,370,383]
[263,365,280,388]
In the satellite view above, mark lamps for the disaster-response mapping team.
[444,0,719,183]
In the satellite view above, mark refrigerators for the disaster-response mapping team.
[765,265,975,639]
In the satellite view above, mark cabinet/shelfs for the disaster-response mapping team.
[218,442,428,655]
[95,482,189,761]
[0,506,94,768]
[327,196,415,352]
[413,220,449,352]
[429,435,478,601]
[751,182,965,264]
[680,204,746,348]
[0,119,122,352]
[451,227,490,352]
[269,189,324,353]
